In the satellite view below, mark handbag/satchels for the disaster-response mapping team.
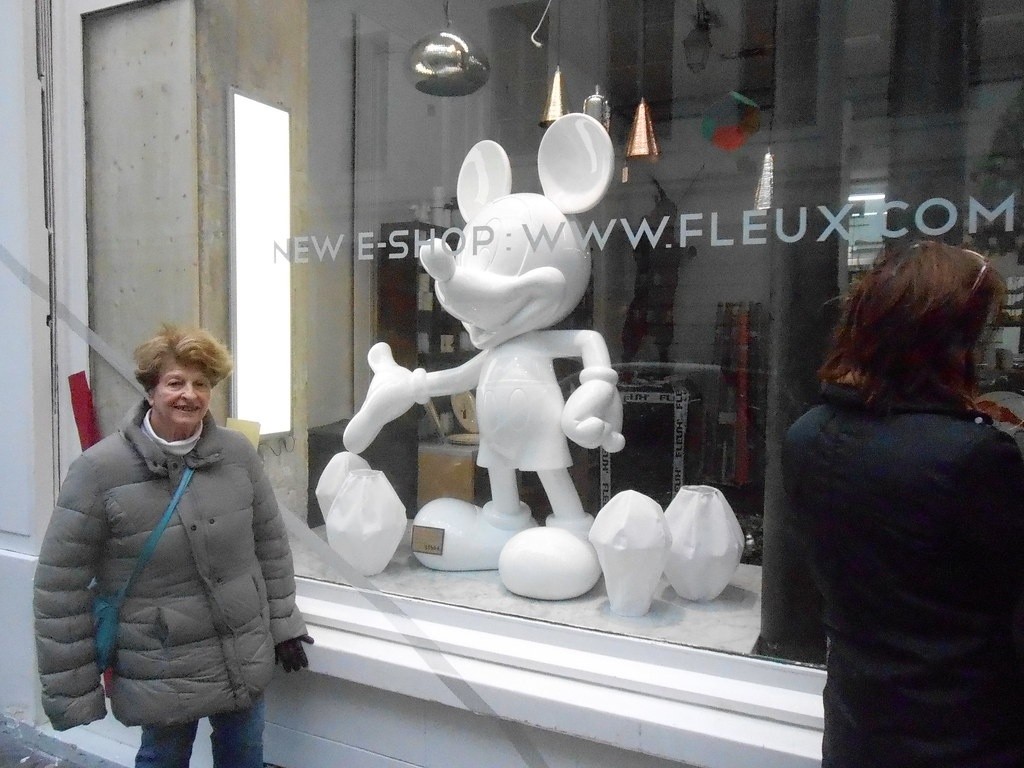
[89,585,118,672]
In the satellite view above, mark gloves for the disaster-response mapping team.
[275,635,314,673]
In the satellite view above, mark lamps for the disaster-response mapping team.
[404,0,784,213]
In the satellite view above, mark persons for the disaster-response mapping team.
[33,320,315,768]
[760,241,1024,768]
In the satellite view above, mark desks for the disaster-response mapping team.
[305,420,526,531]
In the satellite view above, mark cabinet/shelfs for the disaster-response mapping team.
[374,220,595,417]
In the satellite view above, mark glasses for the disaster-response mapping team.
[909,240,989,314]
